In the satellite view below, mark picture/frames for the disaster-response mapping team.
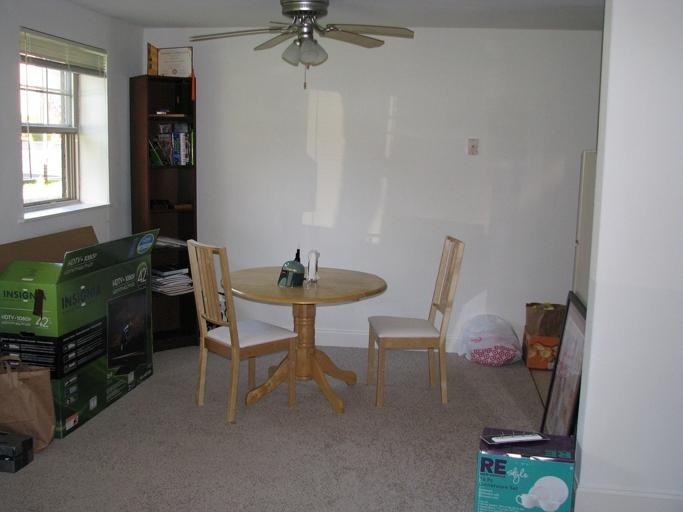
[539,291,587,438]
[156,46,193,78]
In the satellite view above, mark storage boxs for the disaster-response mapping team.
[522,303,566,371]
[473,427,577,512]
[0,225,161,439]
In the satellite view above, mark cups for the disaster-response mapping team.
[515,493,538,509]
[540,500,560,511]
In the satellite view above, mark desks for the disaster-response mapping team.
[220,265,388,415]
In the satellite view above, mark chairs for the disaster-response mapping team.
[366,236,465,409]
[185,238,299,425]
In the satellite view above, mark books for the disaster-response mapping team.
[147,121,195,167]
[150,231,195,296]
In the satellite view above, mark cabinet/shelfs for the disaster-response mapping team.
[129,74,198,337]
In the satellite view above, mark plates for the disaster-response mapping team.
[534,475,568,504]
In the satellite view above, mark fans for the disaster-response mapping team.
[188,0,415,51]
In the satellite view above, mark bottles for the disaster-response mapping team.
[188,128,195,166]
[307,250,320,282]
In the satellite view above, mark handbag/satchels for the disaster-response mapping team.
[0,356,56,450]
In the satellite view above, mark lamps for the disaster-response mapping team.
[281,23,332,91]
